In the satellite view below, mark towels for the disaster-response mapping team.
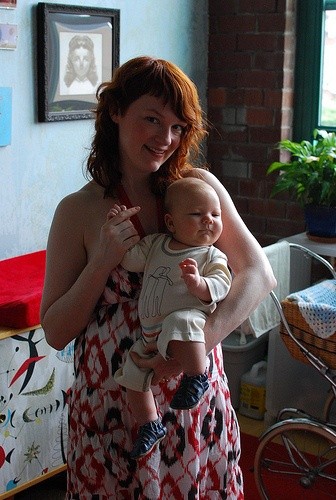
[240,240,291,345]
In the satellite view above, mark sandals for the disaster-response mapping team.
[131,420,167,458]
[170,374,209,410]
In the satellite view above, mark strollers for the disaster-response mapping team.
[253,242,336,500]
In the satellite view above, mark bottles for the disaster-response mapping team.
[239,361,267,419]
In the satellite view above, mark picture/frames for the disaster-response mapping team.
[37,2,120,122]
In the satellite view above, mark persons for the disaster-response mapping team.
[105,177,232,462]
[37,54,280,500]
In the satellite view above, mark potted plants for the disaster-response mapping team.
[266,129,336,244]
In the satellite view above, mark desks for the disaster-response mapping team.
[264,230,336,423]
[0,323,75,500]
